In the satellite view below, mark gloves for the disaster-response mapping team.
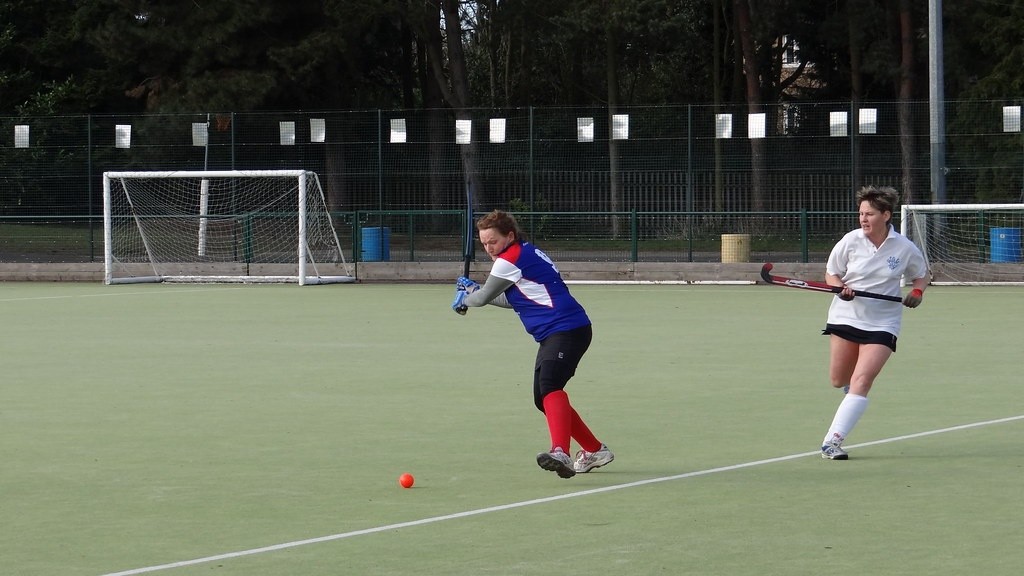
[456,276,480,294]
[902,289,922,308]
[452,292,469,315]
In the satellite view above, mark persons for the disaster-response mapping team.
[820,186,931,459]
[456,210,614,480]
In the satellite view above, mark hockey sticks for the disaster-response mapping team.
[760,263,903,303]
[456,181,473,315]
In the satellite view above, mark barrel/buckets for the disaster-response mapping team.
[362,227,392,262]
[990,227,1023,264]
[720,233,751,263]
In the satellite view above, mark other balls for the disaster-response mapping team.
[399,473,414,488]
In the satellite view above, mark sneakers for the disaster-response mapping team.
[536,447,576,479]
[822,433,848,460]
[574,443,614,472]
[843,384,850,394]
